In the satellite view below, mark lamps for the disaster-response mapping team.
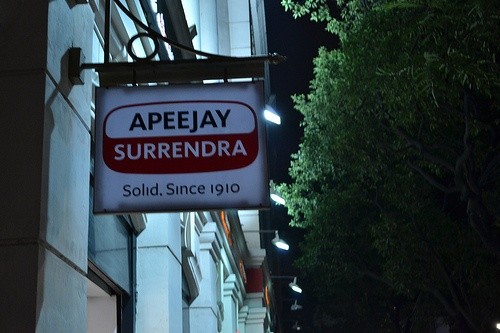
[263,104,281,126]
[188,24,197,39]
[281,319,301,330]
[277,298,302,310]
[270,274,303,294]
[270,191,286,206]
[242,229,290,251]
